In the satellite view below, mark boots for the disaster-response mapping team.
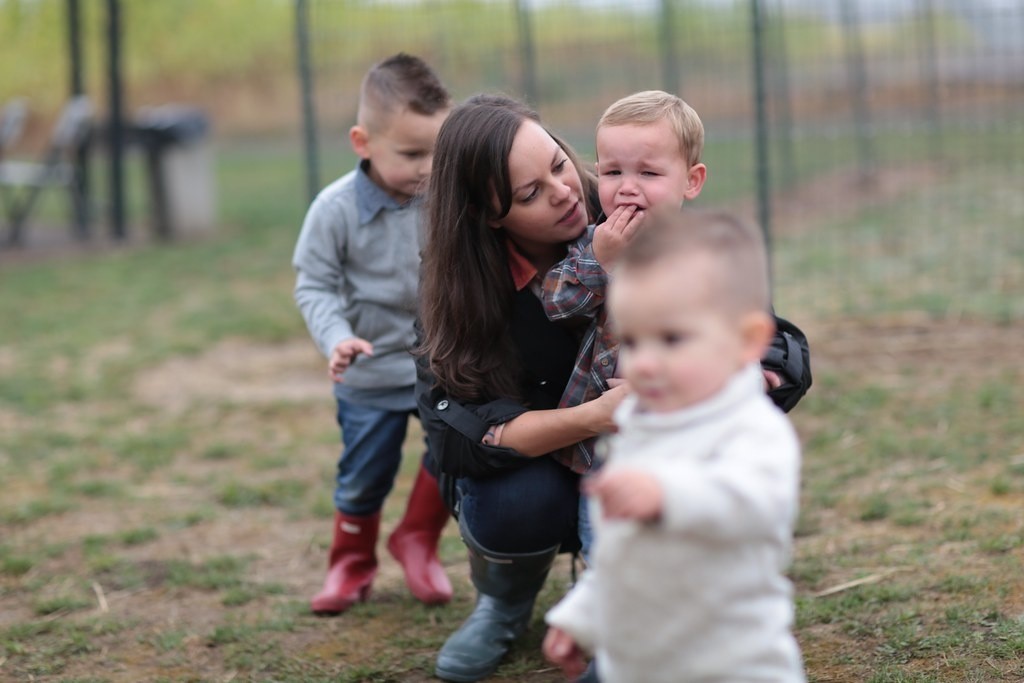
[387,463,453,604]
[574,661,596,683]
[436,494,562,682]
[312,510,382,613]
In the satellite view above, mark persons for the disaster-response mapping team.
[291,52,457,612]
[404,91,813,682]
[541,207,807,682]
[540,92,783,683]
